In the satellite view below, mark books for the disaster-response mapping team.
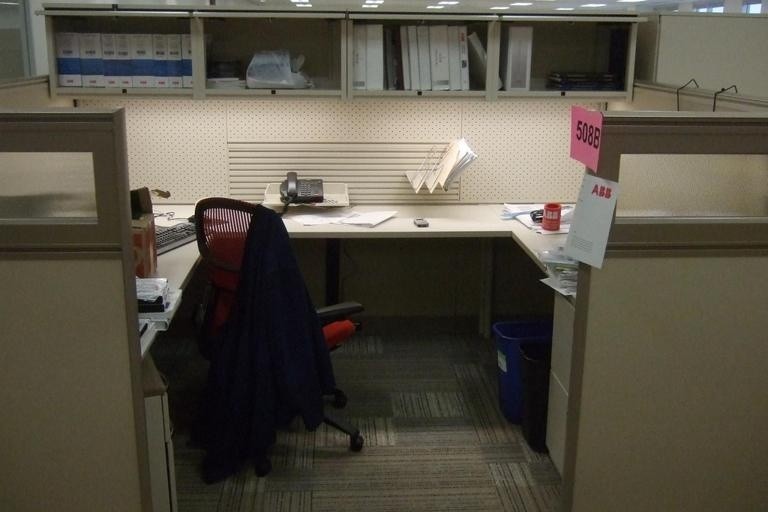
[551,71,613,78]
[546,75,616,83]
[352,21,535,92]
[550,82,619,87]
[136,276,184,338]
[545,85,616,91]
[535,245,578,296]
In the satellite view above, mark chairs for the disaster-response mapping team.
[154,196,365,477]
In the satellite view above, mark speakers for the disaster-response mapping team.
[130,186,154,219]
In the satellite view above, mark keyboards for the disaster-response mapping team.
[154,220,213,256]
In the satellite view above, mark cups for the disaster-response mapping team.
[542,202,561,230]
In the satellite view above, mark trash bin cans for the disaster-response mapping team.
[493,321,553,452]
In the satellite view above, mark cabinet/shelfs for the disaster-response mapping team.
[199,12,340,91]
[499,19,630,94]
[51,10,193,91]
[350,18,489,93]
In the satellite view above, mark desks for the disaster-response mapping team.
[136,202,584,511]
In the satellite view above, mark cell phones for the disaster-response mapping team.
[413,218,429,227]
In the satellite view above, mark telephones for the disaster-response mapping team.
[280,172,323,203]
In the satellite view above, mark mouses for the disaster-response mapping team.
[187,213,209,224]
